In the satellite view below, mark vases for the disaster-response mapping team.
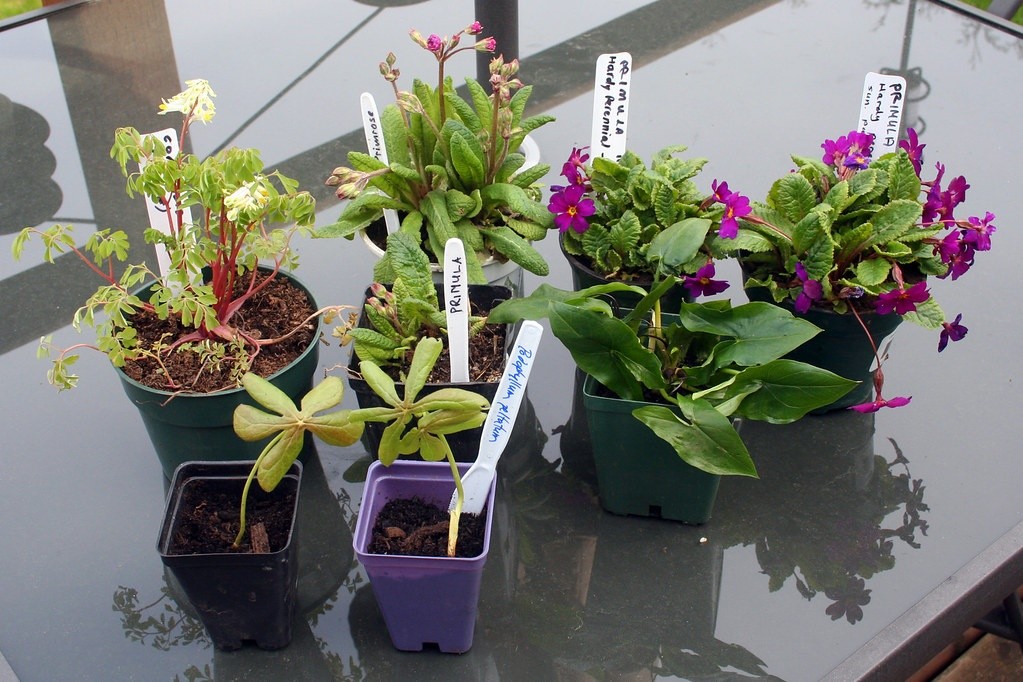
[112,262,320,481]
[738,247,928,411]
[359,228,524,360]
[559,228,697,314]
[508,129,541,183]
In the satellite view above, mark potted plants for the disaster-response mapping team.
[346,334,498,653]
[484,217,863,523]
[157,373,365,652]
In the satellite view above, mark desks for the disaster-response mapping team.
[0,0,1023,682]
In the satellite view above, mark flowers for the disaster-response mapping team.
[311,21,561,286]
[547,130,996,414]
[14,77,357,406]
